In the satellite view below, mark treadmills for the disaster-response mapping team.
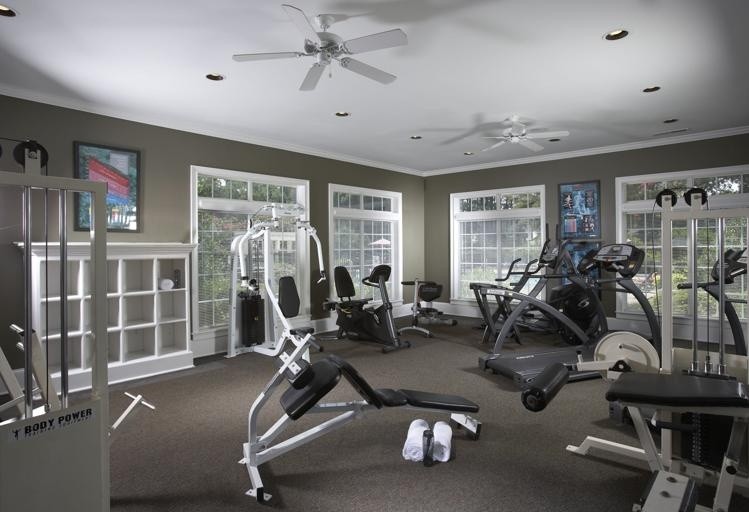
[479,244,662,389]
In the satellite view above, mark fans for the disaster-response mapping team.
[232,4,409,91]
[482,121,570,152]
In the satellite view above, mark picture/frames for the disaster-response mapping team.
[558,180,602,304]
[72,140,141,233]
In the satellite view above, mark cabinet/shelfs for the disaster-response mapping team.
[13,240,196,400]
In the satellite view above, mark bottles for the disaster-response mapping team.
[174,269,181,289]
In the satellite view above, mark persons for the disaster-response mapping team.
[563,190,594,231]
[105,203,131,225]
